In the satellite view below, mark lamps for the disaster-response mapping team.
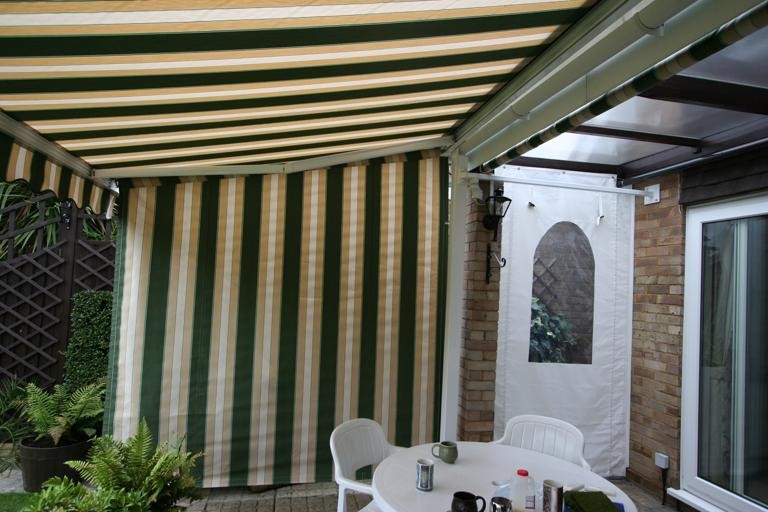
[483,186,511,230]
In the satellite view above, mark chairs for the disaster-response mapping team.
[329,416,407,512]
[487,414,590,469]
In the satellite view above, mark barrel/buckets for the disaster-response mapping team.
[19,430,89,493]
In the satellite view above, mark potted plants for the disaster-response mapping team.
[62,414,211,512]
[18,381,107,492]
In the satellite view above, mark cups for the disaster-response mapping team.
[416,460,433,491]
[430,441,459,465]
[452,491,486,512]
